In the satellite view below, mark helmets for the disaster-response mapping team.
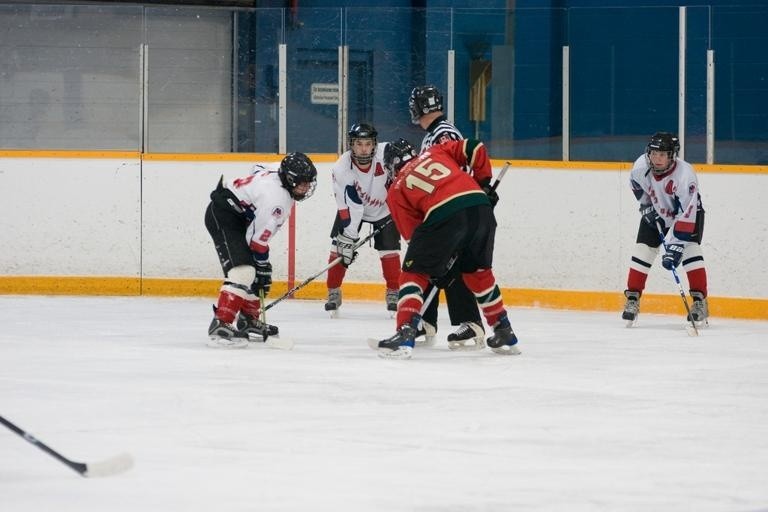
[278,151,317,183]
[410,84,444,117]
[646,130,681,150]
[346,122,377,139]
[383,137,416,166]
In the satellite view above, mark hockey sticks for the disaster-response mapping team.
[655,219,698,337]
[213,219,393,321]
[0,418,134,478]
[248,288,268,342]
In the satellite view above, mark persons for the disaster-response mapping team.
[623,132,707,327]
[324,123,403,310]
[205,153,317,348]
[408,84,486,348]
[377,138,518,360]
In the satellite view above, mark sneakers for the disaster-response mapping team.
[235,309,278,336]
[385,291,398,312]
[486,319,518,349]
[622,289,641,320]
[687,289,709,322]
[417,318,439,337]
[378,325,416,350]
[446,319,486,342]
[324,287,342,311]
[207,316,250,340]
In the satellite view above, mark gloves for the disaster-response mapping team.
[429,266,455,289]
[661,242,684,271]
[335,231,360,267]
[638,203,665,231]
[478,178,500,207]
[253,252,273,299]
